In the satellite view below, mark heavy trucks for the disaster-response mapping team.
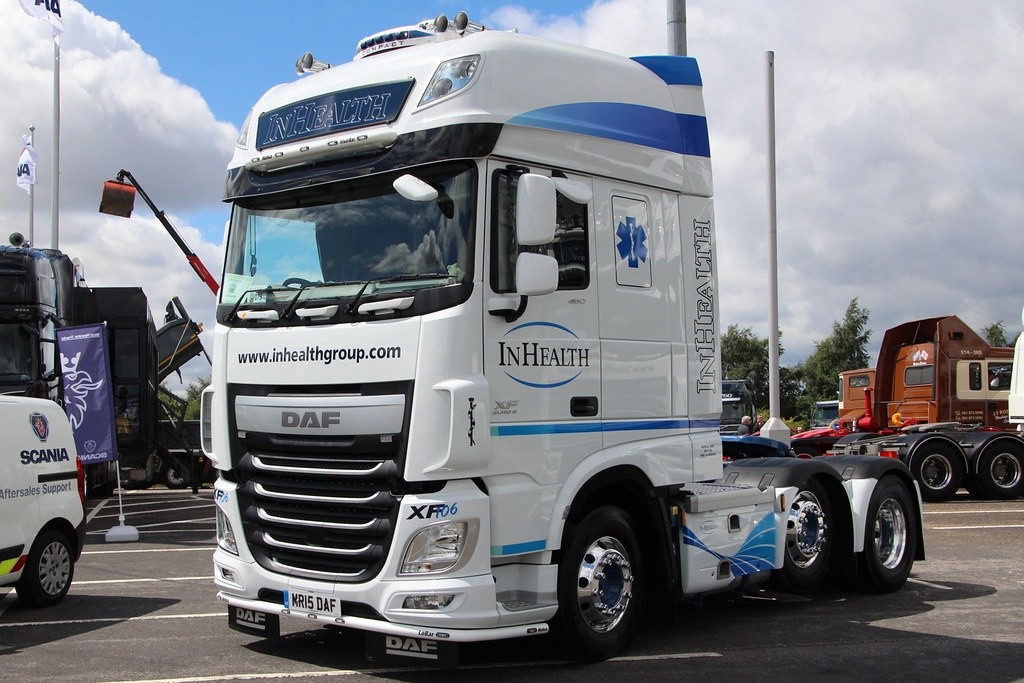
[197,11,929,668]
[0,233,221,503]
[719,314,1024,503]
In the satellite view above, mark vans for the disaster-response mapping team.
[0,393,88,609]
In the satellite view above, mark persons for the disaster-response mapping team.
[737,416,752,435]
[752,415,766,433]
